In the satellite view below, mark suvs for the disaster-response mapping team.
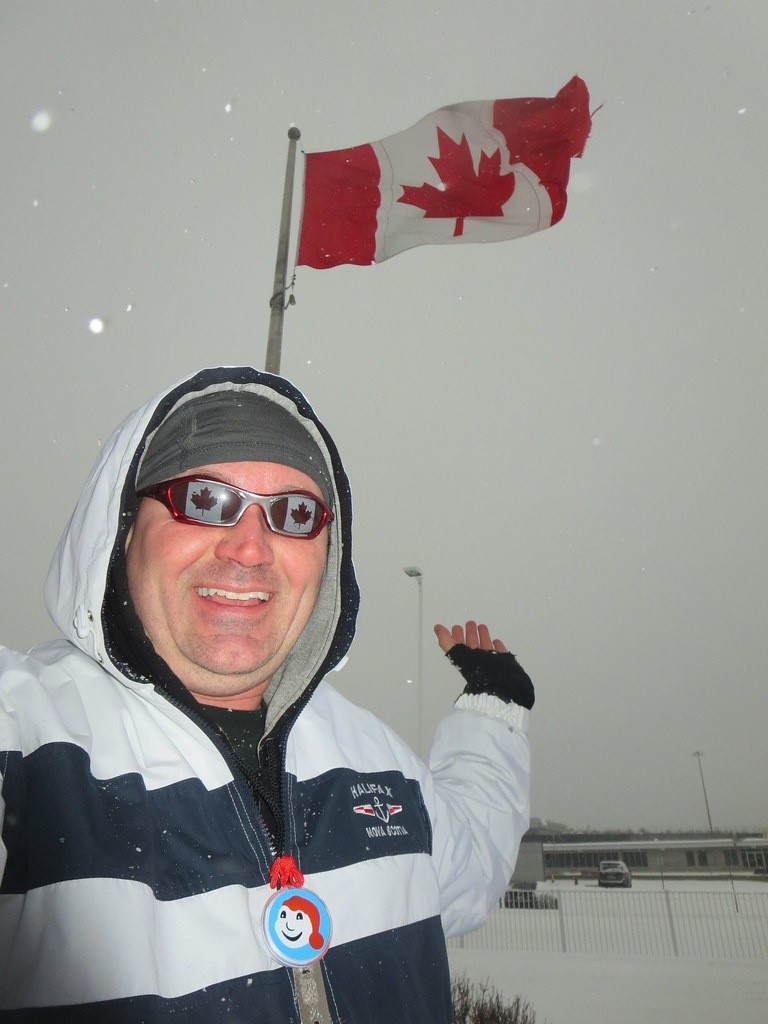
[599,860,632,888]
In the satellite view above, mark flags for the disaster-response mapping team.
[295,72,605,271]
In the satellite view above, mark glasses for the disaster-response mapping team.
[137,474,335,540]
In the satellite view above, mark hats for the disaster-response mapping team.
[136,390,334,534]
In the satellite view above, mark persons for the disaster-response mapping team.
[0,365,536,1023]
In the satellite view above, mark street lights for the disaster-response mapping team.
[692,750,714,832]
[403,567,422,759]
